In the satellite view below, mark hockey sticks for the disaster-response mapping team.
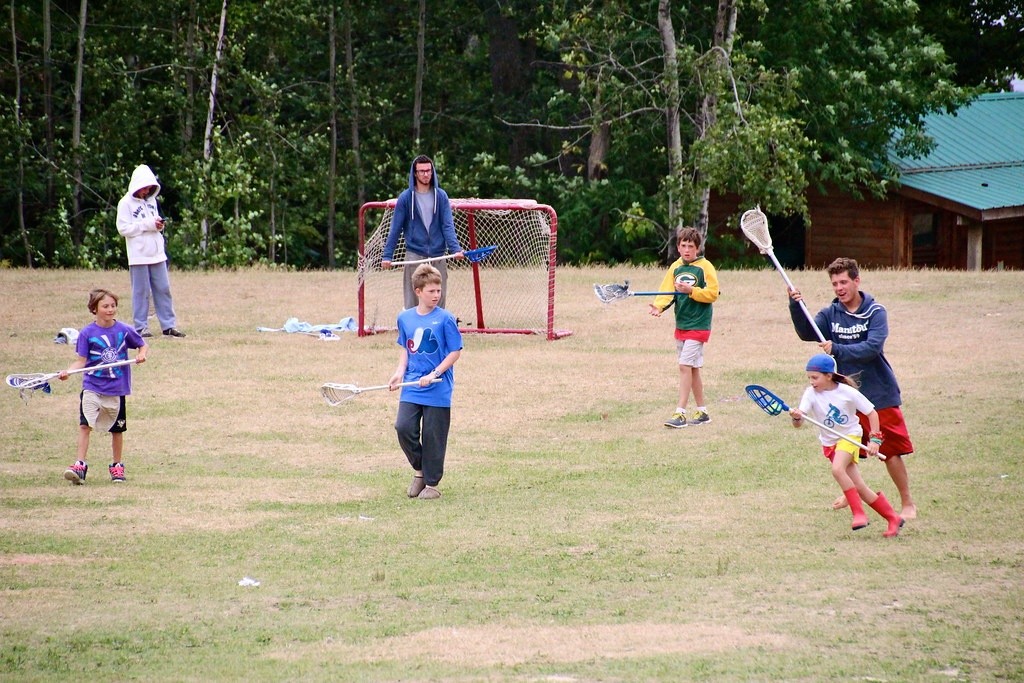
[5,357,147,390]
[321,378,443,406]
[594,283,721,304]
[744,384,887,460]
[390,245,498,267]
[256,326,341,341]
[740,209,835,359]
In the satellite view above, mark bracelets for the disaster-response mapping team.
[432,369,441,378]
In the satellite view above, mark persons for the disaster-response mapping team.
[380,155,464,309]
[787,257,916,520]
[115,164,186,337]
[649,226,718,428]
[59,290,149,486]
[789,352,906,537]
[389,264,463,498]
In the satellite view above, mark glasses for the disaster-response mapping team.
[415,169,434,176]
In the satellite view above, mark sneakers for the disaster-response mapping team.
[162,328,186,338]
[688,410,712,426]
[137,329,155,337]
[664,411,688,428]
[108,462,127,483]
[64,460,89,485]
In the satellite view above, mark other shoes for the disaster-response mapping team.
[407,475,426,497]
[418,486,441,499]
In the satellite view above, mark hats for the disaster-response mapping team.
[806,354,846,382]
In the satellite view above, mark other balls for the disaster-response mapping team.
[769,403,782,414]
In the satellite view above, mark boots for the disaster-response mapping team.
[868,491,905,537]
[843,488,870,530]
